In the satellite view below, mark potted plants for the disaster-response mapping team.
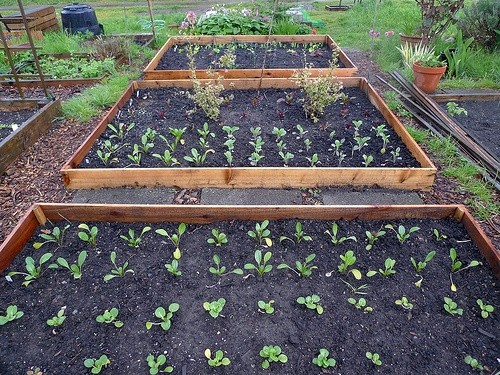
[413,50,447,93]
[400,33,429,52]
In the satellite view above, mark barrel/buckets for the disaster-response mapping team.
[60,2,100,38]
[440,48,456,78]
